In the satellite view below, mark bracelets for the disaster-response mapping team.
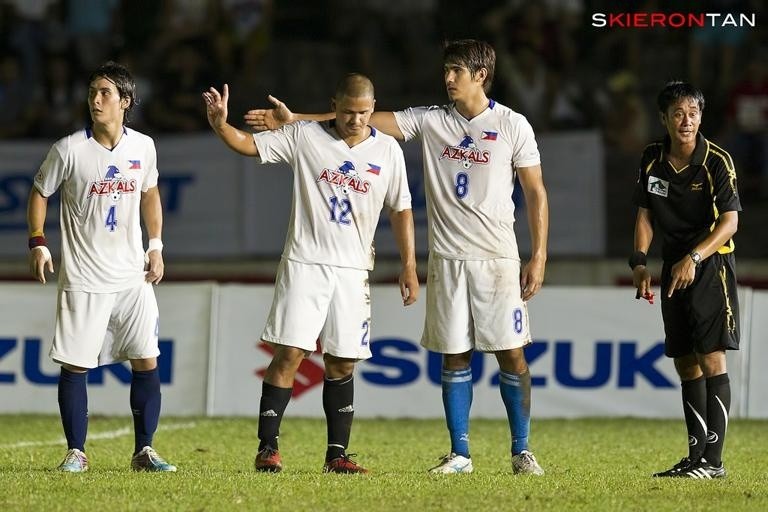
[147,237,166,252]
[628,251,648,270]
[26,229,49,250]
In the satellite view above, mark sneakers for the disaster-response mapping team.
[132,446,177,472]
[57,449,87,472]
[511,449,545,478]
[429,454,473,473]
[322,457,367,474]
[654,459,725,479]
[256,444,281,469]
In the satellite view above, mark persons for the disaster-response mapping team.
[243,36,551,479]
[0,1,767,155]
[24,59,178,477]
[203,72,421,475]
[627,78,745,482]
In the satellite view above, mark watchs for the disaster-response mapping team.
[688,251,701,266]
